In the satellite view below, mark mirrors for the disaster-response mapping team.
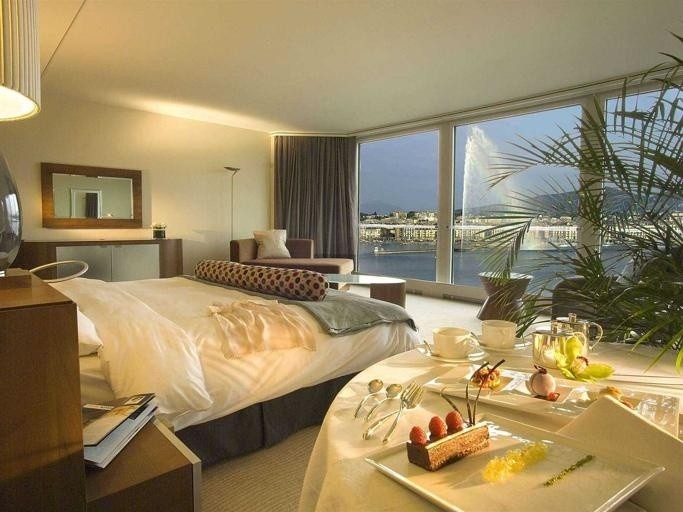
[39,161,144,230]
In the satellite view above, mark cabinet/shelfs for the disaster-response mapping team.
[13,238,184,284]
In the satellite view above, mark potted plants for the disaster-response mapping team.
[471,27,683,376]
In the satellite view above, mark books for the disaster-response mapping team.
[80,392,159,471]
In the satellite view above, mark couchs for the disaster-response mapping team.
[229,237,354,291]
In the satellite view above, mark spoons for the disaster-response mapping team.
[364,383,402,423]
[352,379,384,419]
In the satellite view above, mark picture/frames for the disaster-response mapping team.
[68,186,104,220]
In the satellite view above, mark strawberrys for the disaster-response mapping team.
[428,415,448,436]
[409,426,428,446]
[445,411,463,429]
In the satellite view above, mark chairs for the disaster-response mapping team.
[550,274,636,340]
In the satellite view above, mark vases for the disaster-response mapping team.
[153,228,166,239]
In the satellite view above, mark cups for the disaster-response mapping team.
[433,326,479,358]
[481,319,518,348]
[152,224,166,239]
[556,313,604,350]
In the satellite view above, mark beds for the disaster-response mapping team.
[34,264,423,468]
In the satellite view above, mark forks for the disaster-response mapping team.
[362,381,428,442]
[381,382,424,446]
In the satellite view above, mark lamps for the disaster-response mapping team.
[0,0,42,124]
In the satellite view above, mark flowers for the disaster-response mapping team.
[151,221,168,239]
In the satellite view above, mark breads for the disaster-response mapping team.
[405,409,490,470]
[471,367,501,389]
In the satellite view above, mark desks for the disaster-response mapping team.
[474,270,532,322]
[320,271,407,310]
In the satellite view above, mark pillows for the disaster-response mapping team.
[193,257,328,302]
[72,304,103,359]
[251,227,290,260]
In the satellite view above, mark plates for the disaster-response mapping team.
[555,394,683,512]
[470,334,532,352]
[429,349,490,363]
[360,412,663,511]
[420,361,681,438]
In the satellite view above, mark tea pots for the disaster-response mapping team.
[531,321,587,370]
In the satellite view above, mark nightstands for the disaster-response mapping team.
[0,271,202,511]
